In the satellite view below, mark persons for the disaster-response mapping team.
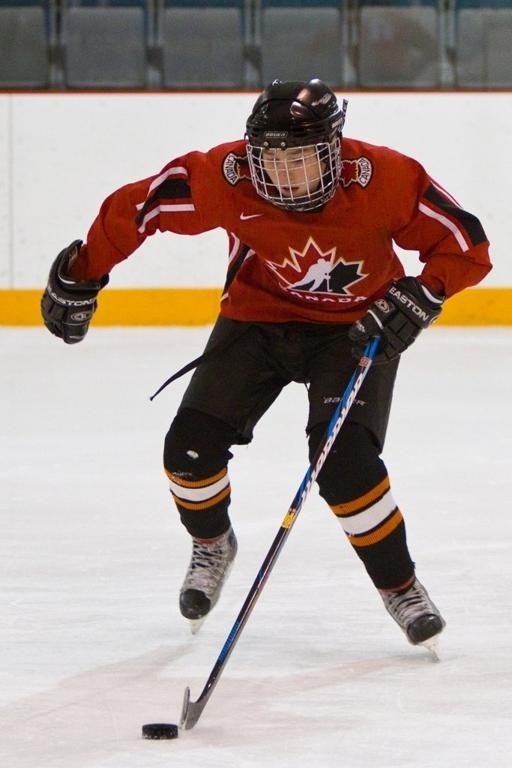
[38,79,491,643]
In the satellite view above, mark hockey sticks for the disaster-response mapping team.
[181,334,383,731]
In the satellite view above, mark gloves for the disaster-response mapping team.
[39,239,111,345]
[348,275,446,368]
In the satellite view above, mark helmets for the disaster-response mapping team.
[243,76,346,159]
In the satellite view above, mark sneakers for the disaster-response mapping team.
[378,578,447,648]
[178,526,239,620]
